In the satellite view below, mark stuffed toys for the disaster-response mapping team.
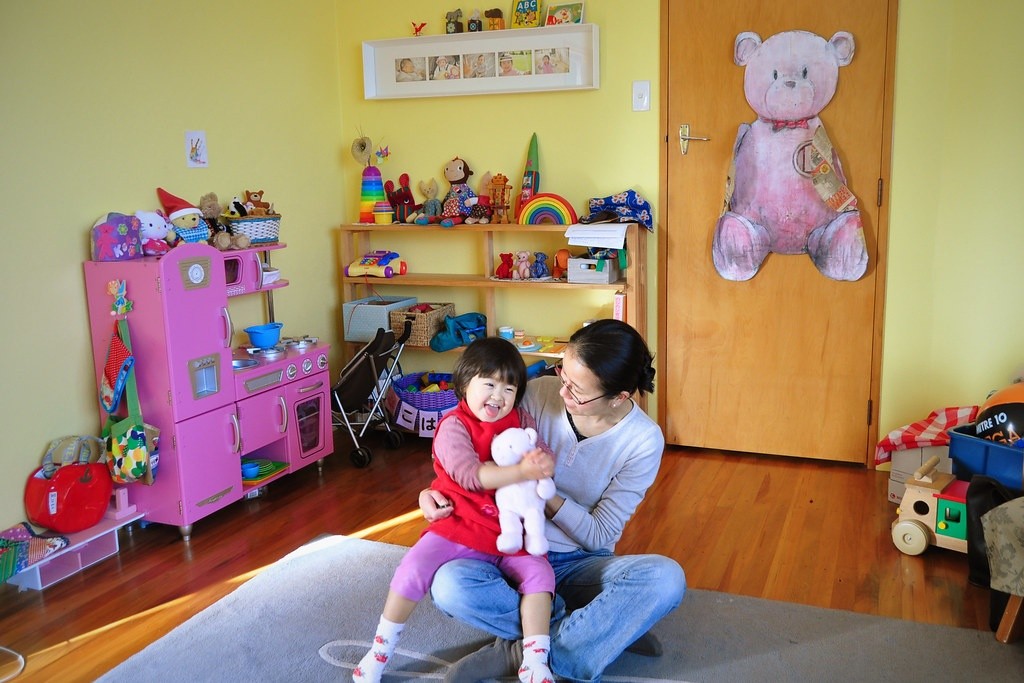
[383,158,573,281]
[135,185,284,257]
[489,426,557,557]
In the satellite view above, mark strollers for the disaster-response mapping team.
[329,314,412,469]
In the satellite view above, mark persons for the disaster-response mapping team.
[538,55,553,74]
[428,318,689,683]
[395,58,423,82]
[350,337,558,683]
[471,55,493,78]
[433,56,460,80]
[498,54,523,75]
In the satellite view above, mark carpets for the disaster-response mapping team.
[91,531,1024,683]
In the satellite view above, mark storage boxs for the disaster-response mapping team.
[222,213,282,245]
[389,302,455,347]
[387,370,460,433]
[946,421,1024,494]
[567,254,621,285]
[344,296,418,342]
[887,444,952,502]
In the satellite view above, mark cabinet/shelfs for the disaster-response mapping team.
[7,506,145,590]
[84,242,243,541]
[361,23,598,101]
[220,242,290,296]
[340,221,648,436]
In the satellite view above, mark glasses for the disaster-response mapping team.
[554,358,610,405]
